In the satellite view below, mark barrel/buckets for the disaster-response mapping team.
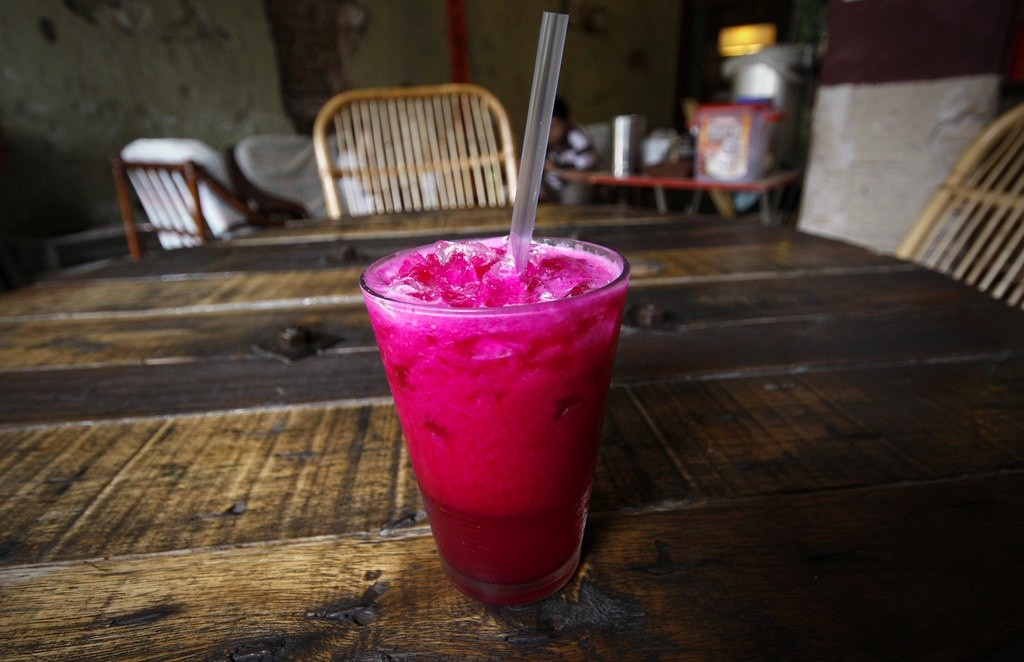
[694,102,788,183]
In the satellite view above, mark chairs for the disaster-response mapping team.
[314,83,518,219]
[112,158,273,255]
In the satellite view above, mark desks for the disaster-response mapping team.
[552,159,796,218]
[0,204,1024,662]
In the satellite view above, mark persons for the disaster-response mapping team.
[539,98,599,206]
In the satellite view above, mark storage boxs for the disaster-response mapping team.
[688,99,788,183]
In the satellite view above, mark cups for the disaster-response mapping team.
[611,114,645,178]
[356,232,631,605]
